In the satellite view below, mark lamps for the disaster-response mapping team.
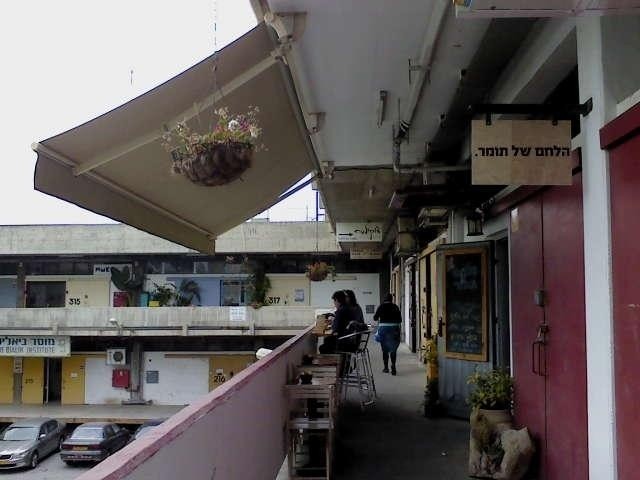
[466,208,483,236]
[109,317,121,335]
[376,90,387,128]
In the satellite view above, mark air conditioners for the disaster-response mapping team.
[394,217,417,258]
[105,348,128,366]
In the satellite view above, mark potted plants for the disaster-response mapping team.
[304,262,329,282]
[424,377,449,419]
[466,364,534,480]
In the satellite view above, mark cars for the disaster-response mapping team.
[0,418,65,470]
[126,419,162,445]
[59,422,130,465]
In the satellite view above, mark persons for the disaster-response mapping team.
[339,289,364,377]
[374,292,402,376]
[318,290,353,352]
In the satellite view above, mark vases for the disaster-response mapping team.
[171,142,252,189]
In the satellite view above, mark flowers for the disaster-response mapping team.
[159,104,270,160]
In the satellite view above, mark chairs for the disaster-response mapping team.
[285,326,375,479]
